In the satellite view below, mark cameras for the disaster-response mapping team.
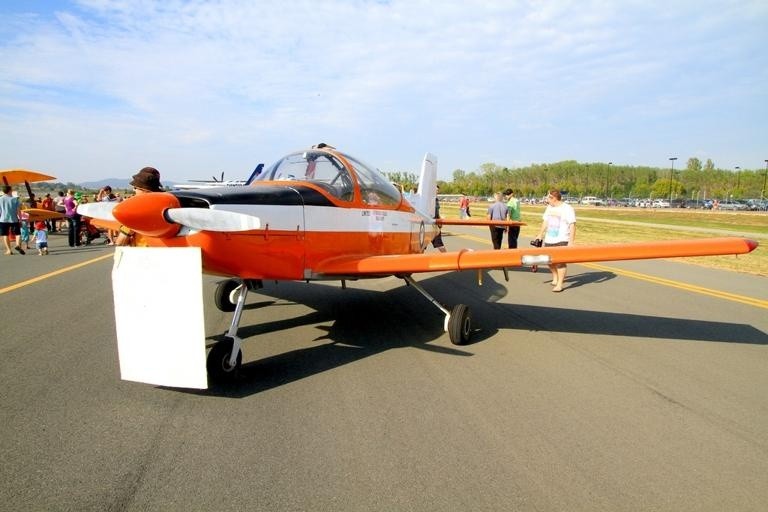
[531,239,543,247]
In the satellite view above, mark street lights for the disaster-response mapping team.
[583,163,589,196]
[667,157,678,202]
[761,159,768,199]
[606,160,613,197]
[734,165,741,188]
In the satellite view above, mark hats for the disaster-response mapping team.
[128,166,165,194]
[502,188,514,195]
[35,221,45,230]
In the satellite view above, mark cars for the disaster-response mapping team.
[518,196,768,212]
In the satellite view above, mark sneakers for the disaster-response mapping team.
[47,228,62,233]
[38,250,48,256]
[106,241,115,246]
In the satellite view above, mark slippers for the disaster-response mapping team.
[4,246,26,256]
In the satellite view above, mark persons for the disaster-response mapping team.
[534,190,576,292]
[578,199,581,207]
[115,166,166,246]
[713,198,719,211]
[604,199,653,208]
[487,192,509,249]
[504,189,521,248]
[367,184,471,252]
[0,184,136,255]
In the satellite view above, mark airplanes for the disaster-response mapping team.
[73,142,759,379]
[0,167,65,233]
[172,171,249,190]
